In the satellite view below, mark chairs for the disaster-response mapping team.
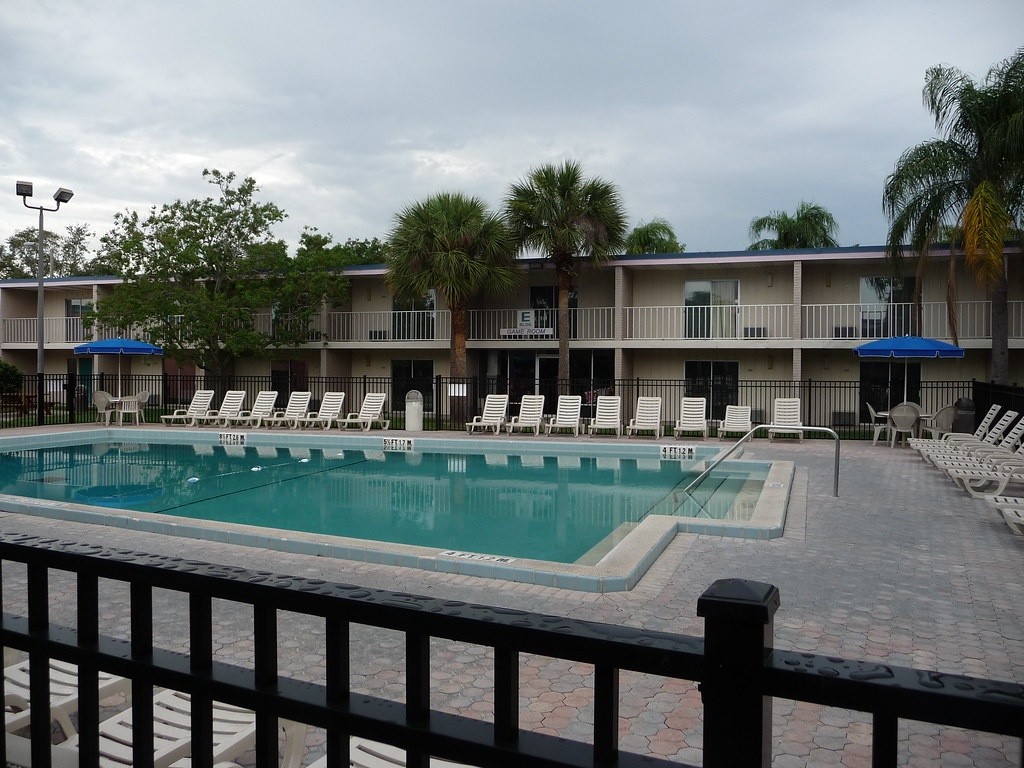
[895,402,921,444]
[336,392,390,432]
[466,394,509,435]
[768,397,803,443]
[92,390,149,426]
[191,445,389,478]
[930,443,1024,536]
[482,454,708,476]
[297,392,345,430]
[889,405,919,448]
[626,396,664,440]
[94,441,149,457]
[866,402,887,445]
[160,390,311,429]
[919,406,956,439]
[588,395,623,437]
[910,411,1019,456]
[673,397,709,440]
[907,404,1001,444]
[717,405,753,442]
[505,395,548,437]
[920,416,1024,464]
[546,395,585,438]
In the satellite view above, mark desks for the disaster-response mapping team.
[107,397,141,425]
[878,411,932,447]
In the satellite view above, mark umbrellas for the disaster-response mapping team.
[73,335,164,401]
[851,334,965,404]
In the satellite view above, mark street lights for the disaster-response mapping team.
[15,182,75,425]
